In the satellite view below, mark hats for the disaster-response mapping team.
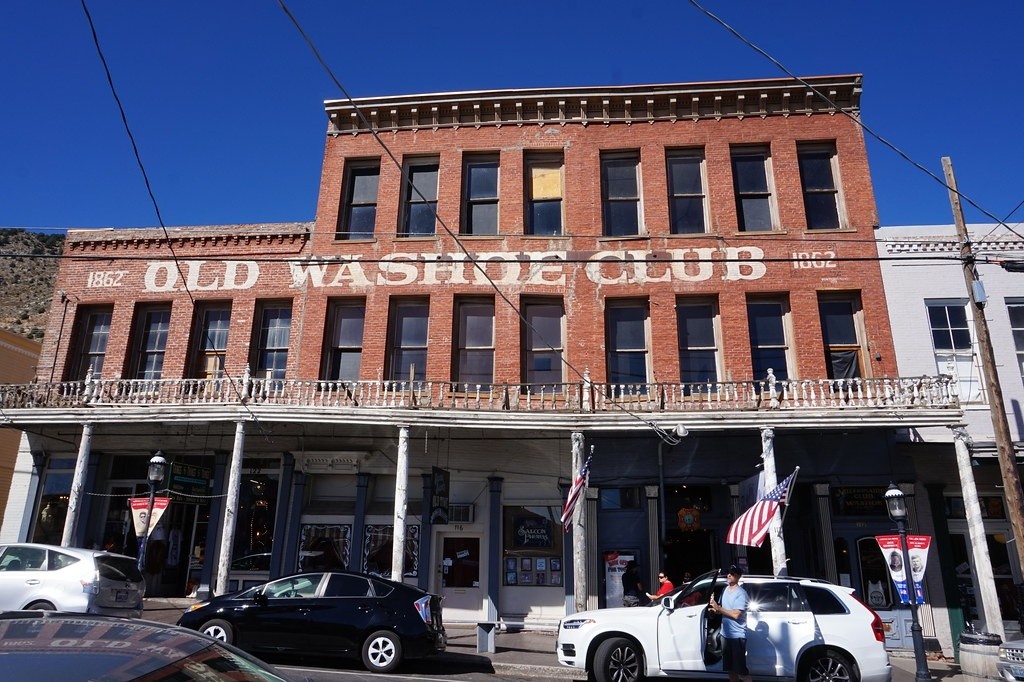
[624,561,640,568]
[725,565,743,575]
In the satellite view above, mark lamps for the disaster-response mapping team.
[253,482,265,495]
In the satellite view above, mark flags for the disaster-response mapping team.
[727,473,792,548]
[560,452,593,533]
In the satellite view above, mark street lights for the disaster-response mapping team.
[882,481,932,682]
[135,448,168,573]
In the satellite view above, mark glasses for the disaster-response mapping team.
[658,576,665,579]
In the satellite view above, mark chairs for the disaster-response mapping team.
[6,560,20,571]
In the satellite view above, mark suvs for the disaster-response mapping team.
[552,568,892,682]
[0,540,147,619]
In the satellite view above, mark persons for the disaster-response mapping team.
[146,512,157,536]
[650,571,673,600]
[678,573,701,607]
[911,555,924,572]
[622,561,643,607]
[135,512,146,536]
[708,564,752,682]
[146,540,166,594]
[890,552,902,572]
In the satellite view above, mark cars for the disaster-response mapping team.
[174,569,448,674]
[0,607,297,682]
